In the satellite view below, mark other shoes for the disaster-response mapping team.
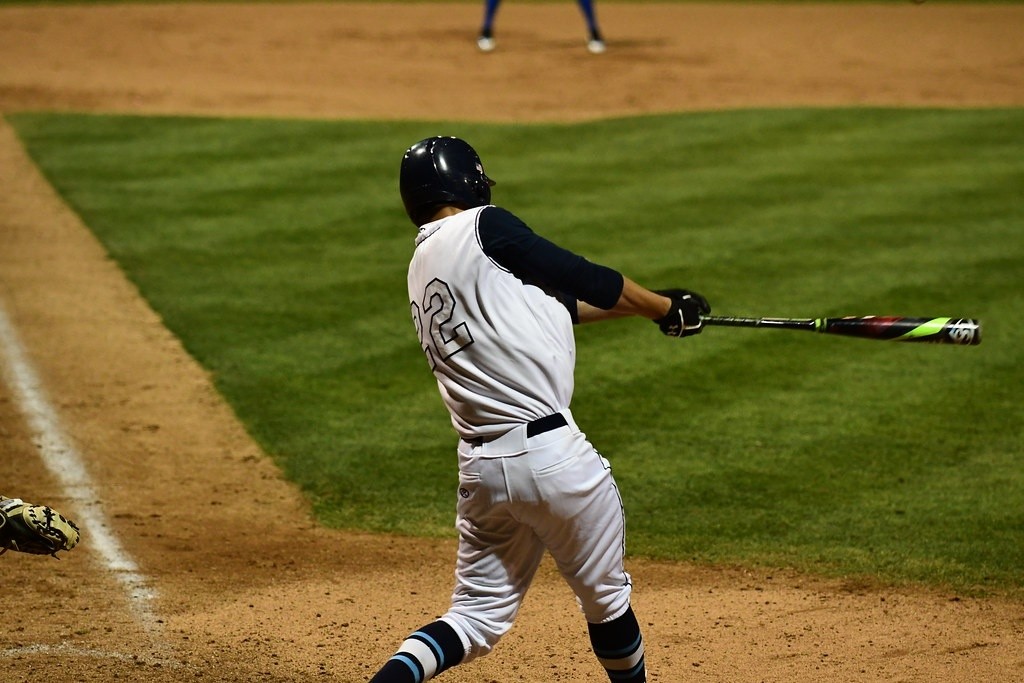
[478,37,492,50]
[587,40,606,55]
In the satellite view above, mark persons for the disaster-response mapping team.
[367,136,711,683]
[476,0,606,55]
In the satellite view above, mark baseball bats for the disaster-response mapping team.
[700,312,982,347]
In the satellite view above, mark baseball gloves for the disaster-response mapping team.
[0,495,81,562]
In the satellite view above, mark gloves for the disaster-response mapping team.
[647,288,711,337]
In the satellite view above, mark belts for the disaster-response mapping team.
[461,412,568,444]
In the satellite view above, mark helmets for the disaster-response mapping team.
[400,135,496,227]
